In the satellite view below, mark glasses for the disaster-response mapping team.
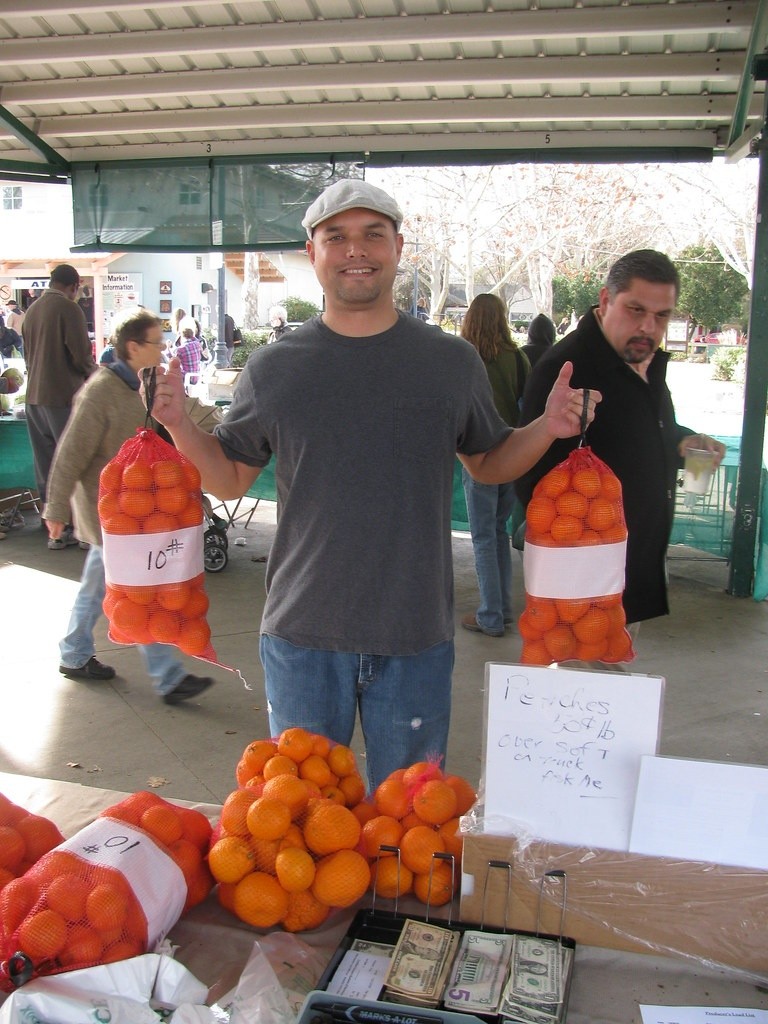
[140,336,163,347]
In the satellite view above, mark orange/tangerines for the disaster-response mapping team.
[519,468,632,665]
[0,790,214,974]
[206,726,371,931]
[353,761,476,907]
[98,457,211,656]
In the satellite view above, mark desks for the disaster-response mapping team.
[0,773,768,1024]
[0,418,768,604]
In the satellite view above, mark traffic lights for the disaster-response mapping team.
[202,283,214,293]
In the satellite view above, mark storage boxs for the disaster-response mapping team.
[458,802,768,974]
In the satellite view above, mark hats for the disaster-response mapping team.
[300,178,403,244]
[6,299,16,306]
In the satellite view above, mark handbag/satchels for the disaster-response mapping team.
[11,344,22,359]
[230,317,243,347]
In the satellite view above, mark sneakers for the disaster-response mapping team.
[164,673,211,704]
[77,541,90,550]
[48,527,78,549]
[58,655,116,680]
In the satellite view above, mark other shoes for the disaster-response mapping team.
[503,617,513,625]
[462,616,481,630]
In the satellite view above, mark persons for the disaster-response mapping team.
[138,178,603,796]
[460,294,532,637]
[513,250,724,672]
[0,289,45,359]
[174,306,292,397]
[517,312,556,369]
[21,265,101,549]
[556,314,585,337]
[410,298,427,322]
[42,312,214,705]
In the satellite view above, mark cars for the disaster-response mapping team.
[692,335,704,343]
[704,333,748,346]
[267,322,303,344]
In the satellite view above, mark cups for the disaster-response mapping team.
[683,448,719,496]
